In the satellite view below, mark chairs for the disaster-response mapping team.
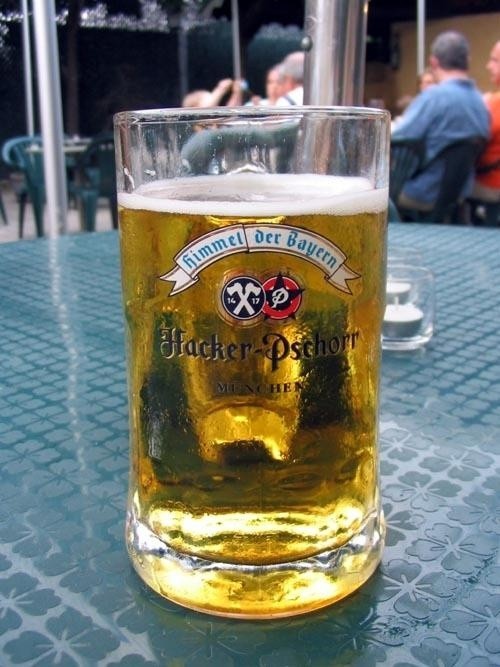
[1,132,118,239]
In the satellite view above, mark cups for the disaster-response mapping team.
[383,246,422,303]
[380,263,435,360]
[114,106,392,622]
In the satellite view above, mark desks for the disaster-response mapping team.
[0,216,500,667]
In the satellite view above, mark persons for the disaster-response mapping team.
[250,62,285,107]
[189,75,246,136]
[274,51,308,110]
[391,31,491,214]
[460,39,500,225]
[418,68,437,94]
